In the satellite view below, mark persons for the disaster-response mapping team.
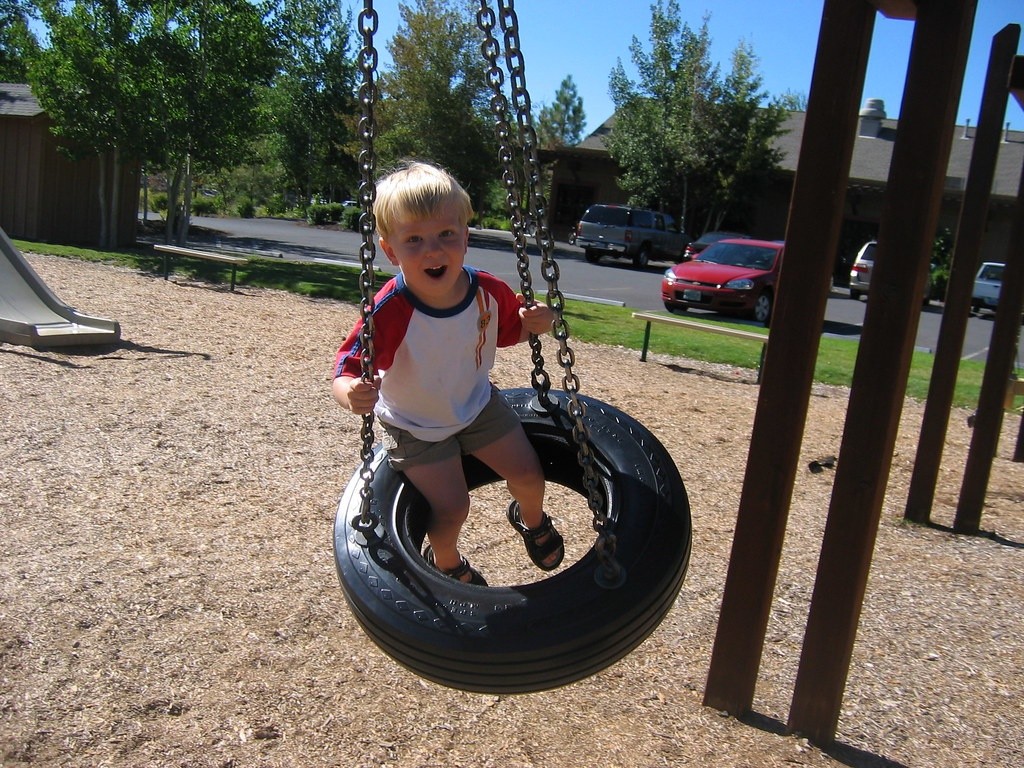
[331,160,564,587]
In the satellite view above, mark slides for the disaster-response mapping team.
[0,225,121,351]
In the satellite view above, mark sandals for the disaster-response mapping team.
[423,545,487,592]
[505,498,565,572]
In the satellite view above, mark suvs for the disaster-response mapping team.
[576,204,692,270]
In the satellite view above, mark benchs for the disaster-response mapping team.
[155,243,248,291]
[632,310,769,385]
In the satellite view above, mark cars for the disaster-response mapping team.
[684,231,752,261]
[343,201,362,207]
[972,263,1006,311]
[849,241,936,306]
[661,238,785,322]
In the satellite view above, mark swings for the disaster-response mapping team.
[328,0,699,700]
